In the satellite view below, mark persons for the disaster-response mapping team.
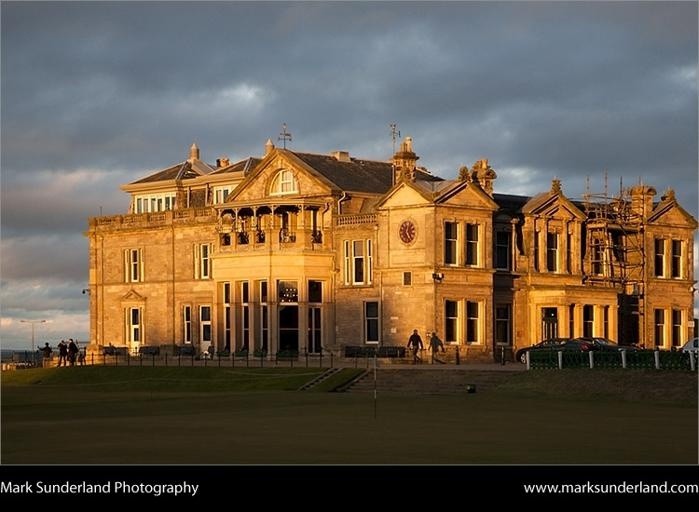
[208,340,214,355]
[67,338,79,366]
[428,332,447,365]
[406,328,425,364]
[108,342,114,355]
[56,340,68,367]
[37,341,53,369]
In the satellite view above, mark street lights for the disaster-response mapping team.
[20,319,47,362]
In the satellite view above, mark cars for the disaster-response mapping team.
[582,337,640,353]
[679,336,699,353]
[516,337,596,364]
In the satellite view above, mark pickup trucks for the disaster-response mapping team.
[8,361,28,367]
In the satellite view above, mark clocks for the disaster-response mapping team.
[396,218,418,247]
